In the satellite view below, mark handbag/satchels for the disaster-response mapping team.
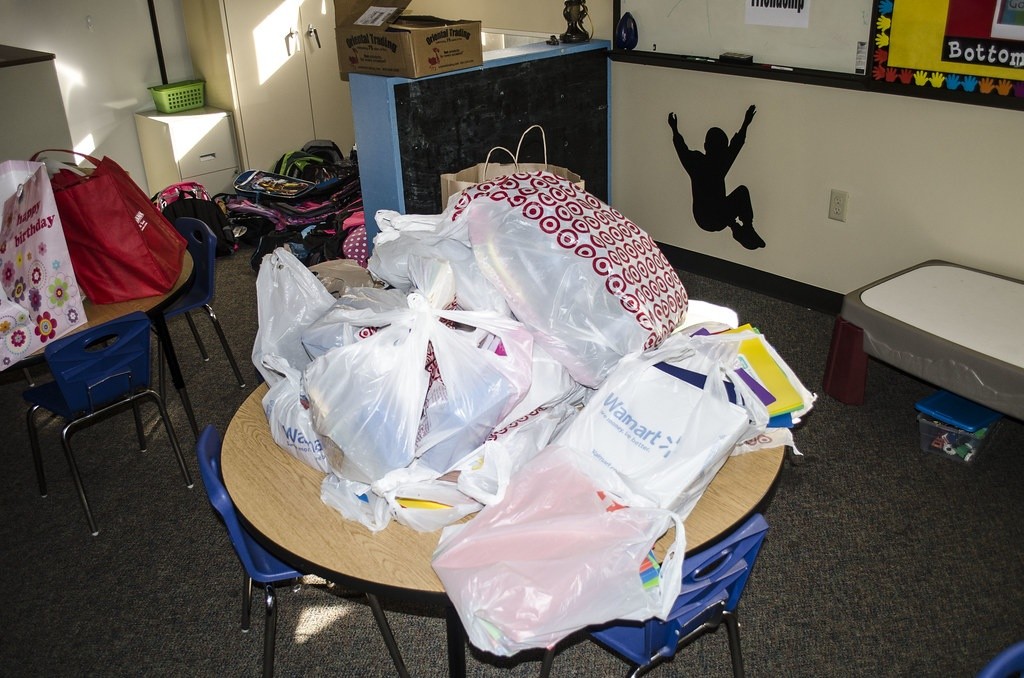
[547,345,769,528]
[251,170,741,533]
[440,125,584,213]
[430,444,686,657]
[28,149,188,305]
[0,160,89,372]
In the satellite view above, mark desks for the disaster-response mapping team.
[823,259,1024,440]
[0,244,204,445]
[219,303,787,678]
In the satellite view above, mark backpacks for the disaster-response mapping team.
[150,139,369,274]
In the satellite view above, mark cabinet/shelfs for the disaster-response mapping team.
[134,104,243,208]
[179,0,354,173]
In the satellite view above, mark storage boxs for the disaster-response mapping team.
[332,0,483,83]
[147,79,206,114]
[913,389,1004,465]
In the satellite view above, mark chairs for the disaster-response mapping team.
[540,511,769,678]
[21,309,194,538]
[195,423,409,678]
[102,216,246,456]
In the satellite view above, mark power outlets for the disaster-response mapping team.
[828,189,849,224]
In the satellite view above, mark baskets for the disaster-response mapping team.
[146,79,206,114]
